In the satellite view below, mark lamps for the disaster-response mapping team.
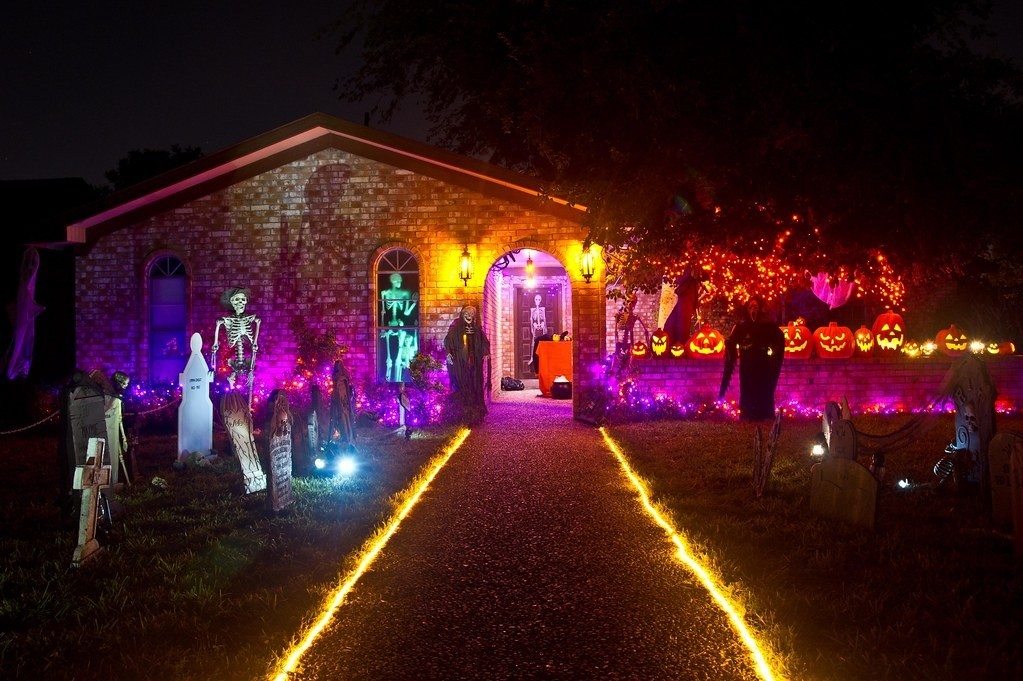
[580,241,594,283]
[458,242,472,287]
[525,248,536,280]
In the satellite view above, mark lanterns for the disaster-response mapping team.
[670,341,686,359]
[872,309,907,357]
[632,340,648,358]
[650,327,670,359]
[813,321,855,358]
[922,338,938,356]
[904,338,923,357]
[936,323,971,357]
[985,339,999,354]
[854,324,874,356]
[684,322,726,359]
[778,321,813,359]
